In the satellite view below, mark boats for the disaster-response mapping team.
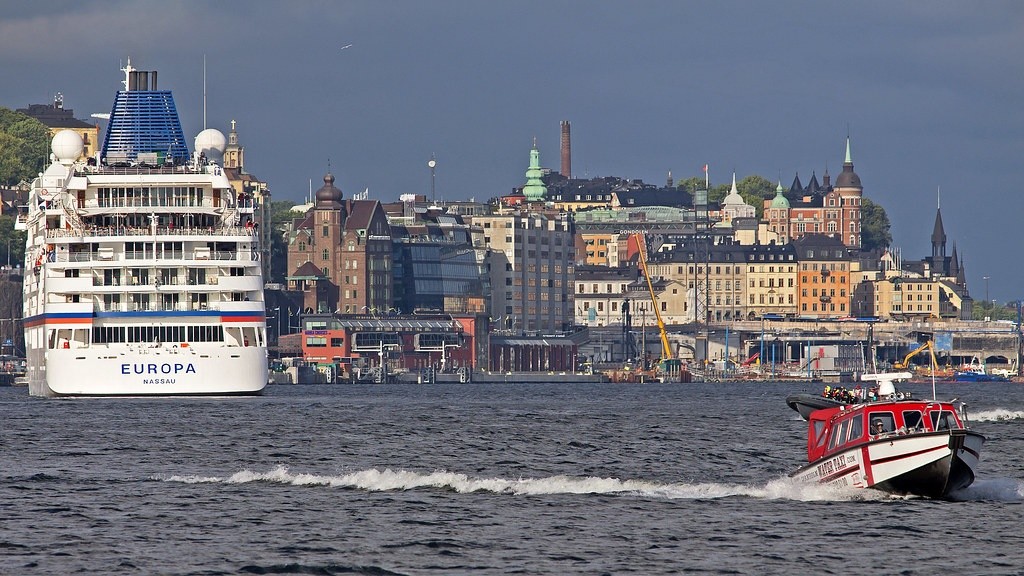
[786,345,926,422]
[788,393,987,500]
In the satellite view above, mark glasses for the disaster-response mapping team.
[878,424,883,426]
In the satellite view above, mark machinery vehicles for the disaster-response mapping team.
[891,339,940,372]
[634,234,681,371]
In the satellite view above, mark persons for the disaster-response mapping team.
[46,246,55,263]
[822,385,877,404]
[244,218,259,236]
[873,419,889,435]
[82,223,136,235]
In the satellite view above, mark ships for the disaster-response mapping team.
[13,53,269,398]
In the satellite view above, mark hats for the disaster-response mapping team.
[877,422,883,425]
